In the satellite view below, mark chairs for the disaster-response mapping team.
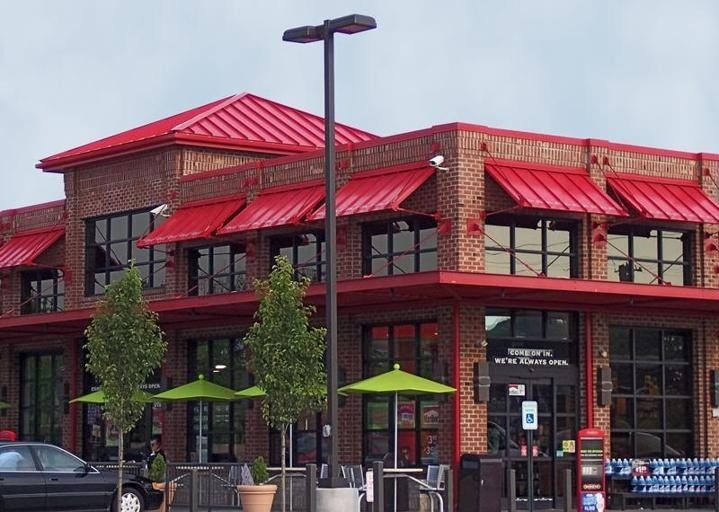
[169,462,450,512]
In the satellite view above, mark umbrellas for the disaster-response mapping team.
[67,387,167,405]
[335,363,459,512]
[149,373,242,508]
[234,384,349,512]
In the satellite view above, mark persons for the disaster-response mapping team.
[0,448,25,472]
[140,432,168,512]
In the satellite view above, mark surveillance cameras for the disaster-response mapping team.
[149,203,170,218]
[428,155,449,171]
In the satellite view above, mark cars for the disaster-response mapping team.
[0,439,164,511]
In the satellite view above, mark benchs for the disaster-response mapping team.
[605,456,719,510]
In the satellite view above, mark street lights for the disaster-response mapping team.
[278,13,380,512]
